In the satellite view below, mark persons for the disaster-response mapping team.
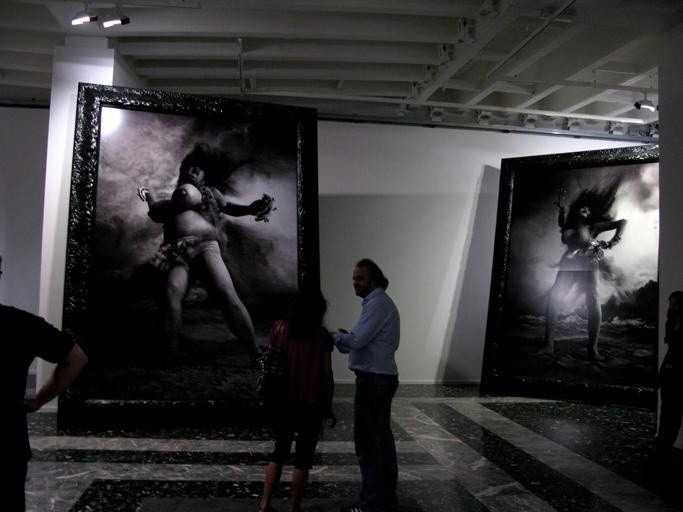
[646,292,683,447]
[257,289,336,512]
[535,176,626,361]
[137,144,276,372]
[0,257,89,512]
[330,259,400,512]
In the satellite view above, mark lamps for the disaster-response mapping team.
[633,90,660,113]
[69,1,129,31]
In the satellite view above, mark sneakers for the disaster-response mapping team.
[644,436,673,448]
[339,504,374,512]
[256,495,270,512]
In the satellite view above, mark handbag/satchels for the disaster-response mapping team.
[256,360,279,404]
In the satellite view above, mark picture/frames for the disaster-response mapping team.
[475,140,658,410]
[53,80,335,441]
